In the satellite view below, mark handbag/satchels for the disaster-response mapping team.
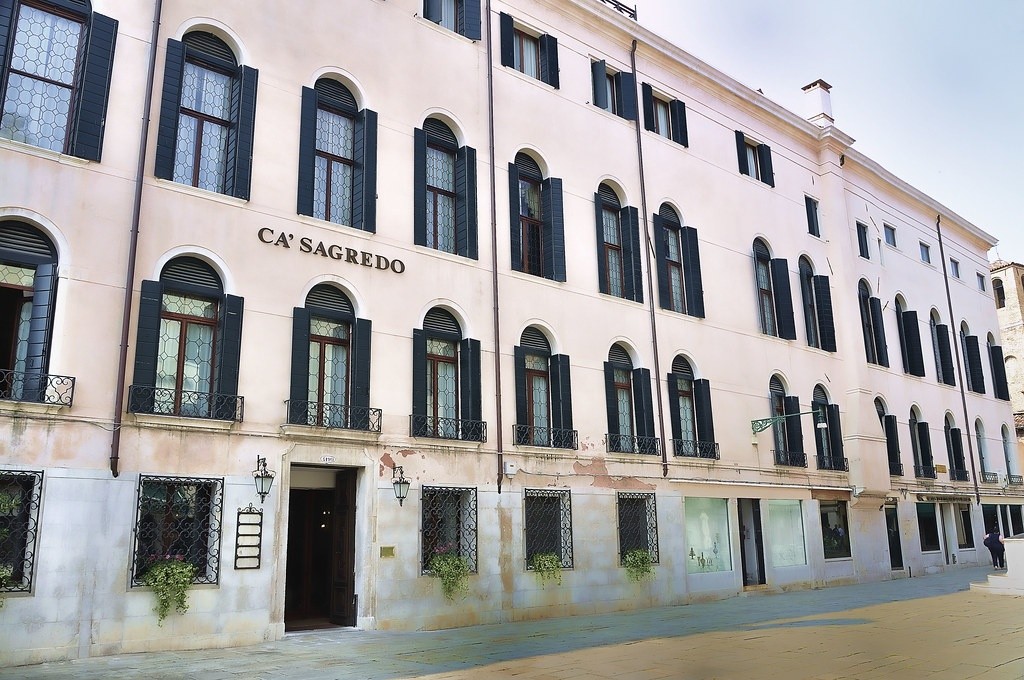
[983,538,993,547]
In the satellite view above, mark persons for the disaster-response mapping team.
[983,527,1005,570]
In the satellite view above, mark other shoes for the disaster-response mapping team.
[995,566,998,570]
[1001,567,1003,569]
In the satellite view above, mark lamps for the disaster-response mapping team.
[391,463,411,507]
[251,455,277,503]
[752,406,827,435]
